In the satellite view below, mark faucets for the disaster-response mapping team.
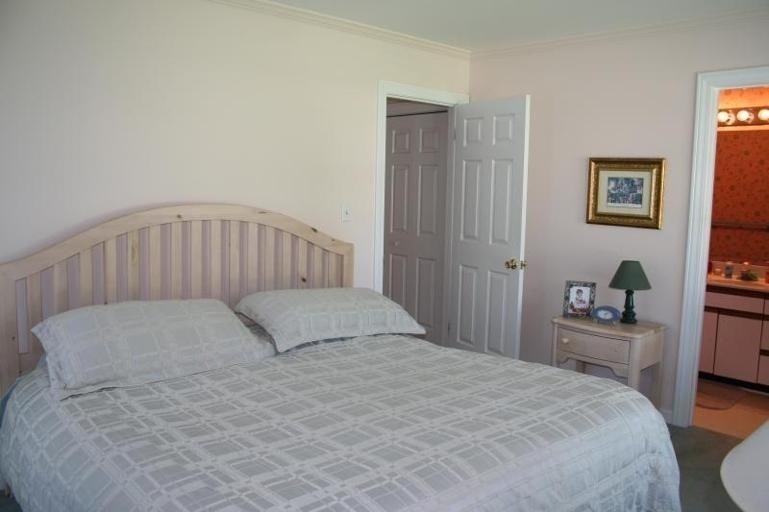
[735,261,763,281]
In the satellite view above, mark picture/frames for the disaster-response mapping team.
[563,280,597,321]
[587,156,665,230]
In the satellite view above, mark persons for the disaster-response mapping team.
[575,289,586,306]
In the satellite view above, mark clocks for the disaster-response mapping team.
[592,306,621,325]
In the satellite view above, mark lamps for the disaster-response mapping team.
[607,260,652,324]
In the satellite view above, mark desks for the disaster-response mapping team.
[551,316,666,409]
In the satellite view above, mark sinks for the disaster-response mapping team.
[709,279,769,288]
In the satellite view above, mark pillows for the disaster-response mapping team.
[234,288,427,351]
[30,298,277,402]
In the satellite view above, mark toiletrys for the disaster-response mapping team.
[724,261,735,279]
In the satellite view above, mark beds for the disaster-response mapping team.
[1,202,681,511]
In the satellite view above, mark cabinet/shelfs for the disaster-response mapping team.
[699,276,768,396]
